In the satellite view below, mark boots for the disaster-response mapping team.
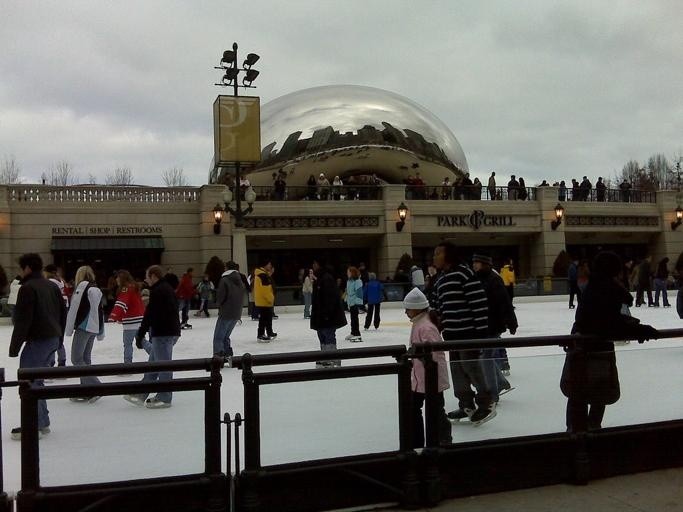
[345,334,354,341]
[663,303,671,308]
[252,315,261,321]
[500,362,512,377]
[272,314,278,319]
[305,313,312,320]
[319,359,341,368]
[636,302,647,308]
[12,426,44,440]
[87,394,104,406]
[496,378,515,397]
[654,303,660,308]
[375,327,378,330]
[257,334,271,344]
[316,360,323,368]
[648,302,655,307]
[469,399,498,428]
[146,393,172,409]
[446,400,477,426]
[39,426,50,434]
[212,350,234,369]
[182,324,193,330]
[348,335,362,342]
[569,305,575,309]
[68,397,89,403]
[124,390,149,406]
[268,332,277,341]
[364,327,369,330]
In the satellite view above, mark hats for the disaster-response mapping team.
[319,173,325,178]
[471,245,493,265]
[403,285,430,310]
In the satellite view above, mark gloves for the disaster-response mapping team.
[136,335,146,350]
[638,324,658,343]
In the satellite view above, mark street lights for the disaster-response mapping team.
[212,41,262,315]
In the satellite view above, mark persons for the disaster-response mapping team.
[1,255,683,342]
[425,242,518,423]
[310,258,347,366]
[404,172,607,202]
[108,270,153,363]
[274,175,286,201]
[123,265,181,409]
[303,172,382,200]
[9,252,68,440]
[560,259,660,432]
[222,173,250,201]
[213,262,244,357]
[403,287,452,445]
[66,266,104,404]
[620,179,632,202]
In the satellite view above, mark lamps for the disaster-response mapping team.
[671,205,683,231]
[221,184,256,228]
[551,203,565,230]
[395,201,409,233]
[213,202,223,237]
